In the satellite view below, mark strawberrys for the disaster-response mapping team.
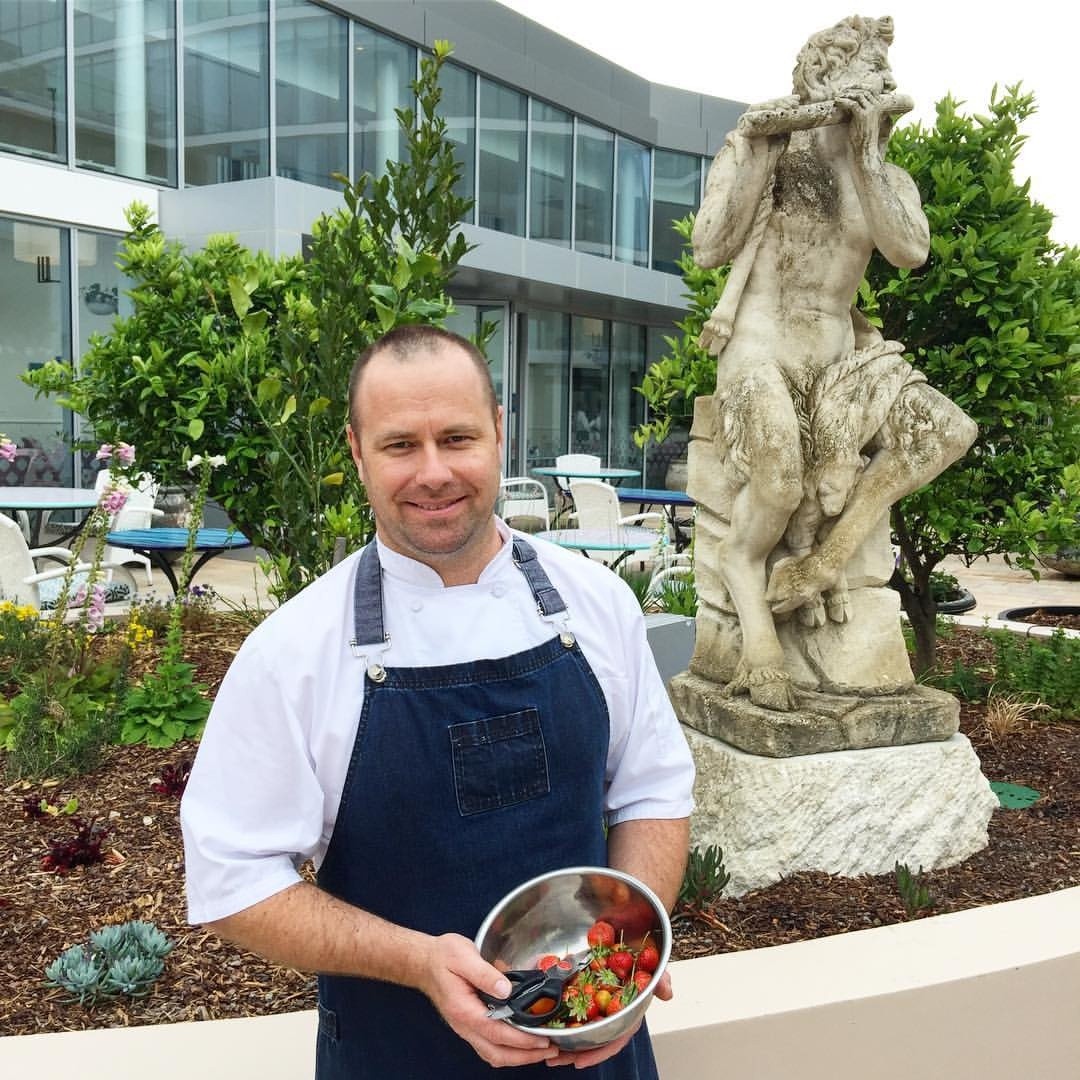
[530,920,658,1030]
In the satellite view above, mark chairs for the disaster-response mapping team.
[0,436,162,622]
[492,454,704,617]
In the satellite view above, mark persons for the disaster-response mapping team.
[575,403,590,448]
[180,325,696,1080]
[690,14,978,712]
[590,411,601,446]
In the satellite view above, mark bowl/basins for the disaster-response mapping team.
[475,865,673,1052]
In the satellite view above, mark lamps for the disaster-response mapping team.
[13,221,98,283]
[79,282,119,316]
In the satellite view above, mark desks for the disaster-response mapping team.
[562,487,696,572]
[0,486,102,575]
[529,466,641,531]
[101,527,252,598]
[536,526,668,571]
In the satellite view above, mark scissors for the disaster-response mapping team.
[477,947,599,1027]
